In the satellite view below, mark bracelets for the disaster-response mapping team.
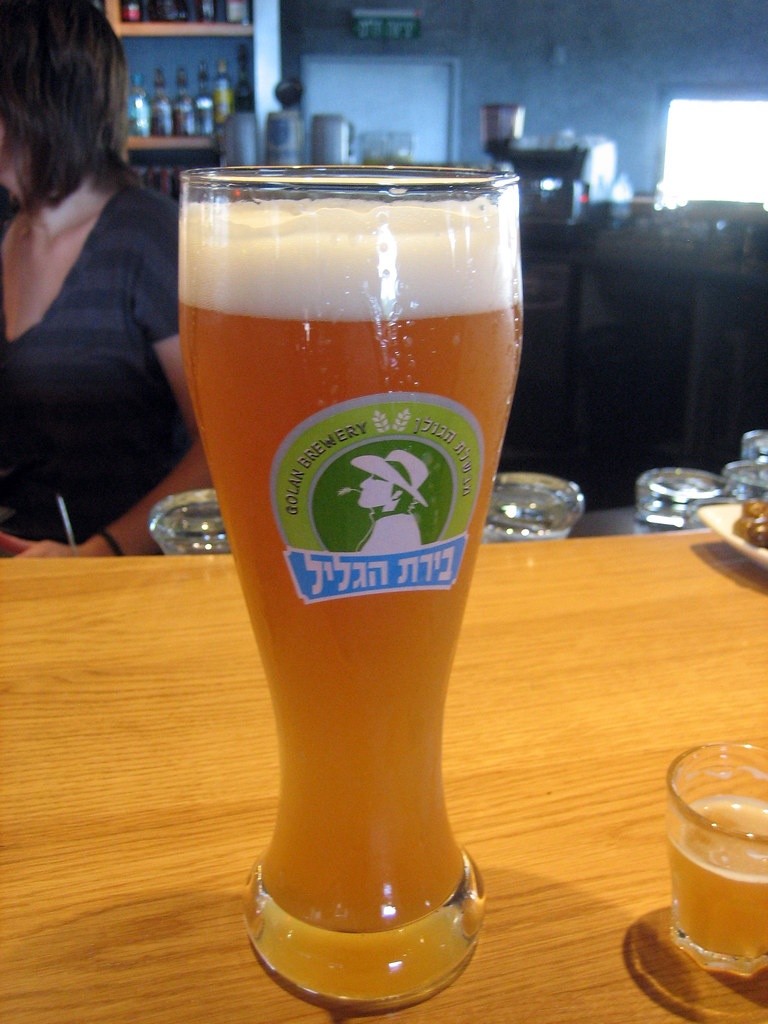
[94,528,124,561]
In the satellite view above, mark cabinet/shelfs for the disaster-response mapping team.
[105,0,253,163]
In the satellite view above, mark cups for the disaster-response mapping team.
[665,742,768,979]
[147,488,232,555]
[359,130,413,166]
[631,466,729,533]
[172,162,532,1013]
[739,428,768,464]
[722,460,768,546]
[477,472,585,544]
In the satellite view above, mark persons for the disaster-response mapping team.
[0,0,214,559]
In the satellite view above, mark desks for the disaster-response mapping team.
[0,529,768,1024]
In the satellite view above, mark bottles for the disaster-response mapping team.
[149,67,174,137]
[128,69,151,136]
[172,66,198,137]
[194,63,214,136]
[213,56,235,130]
[119,0,246,22]
[234,43,252,115]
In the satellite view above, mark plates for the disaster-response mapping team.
[696,504,768,569]
